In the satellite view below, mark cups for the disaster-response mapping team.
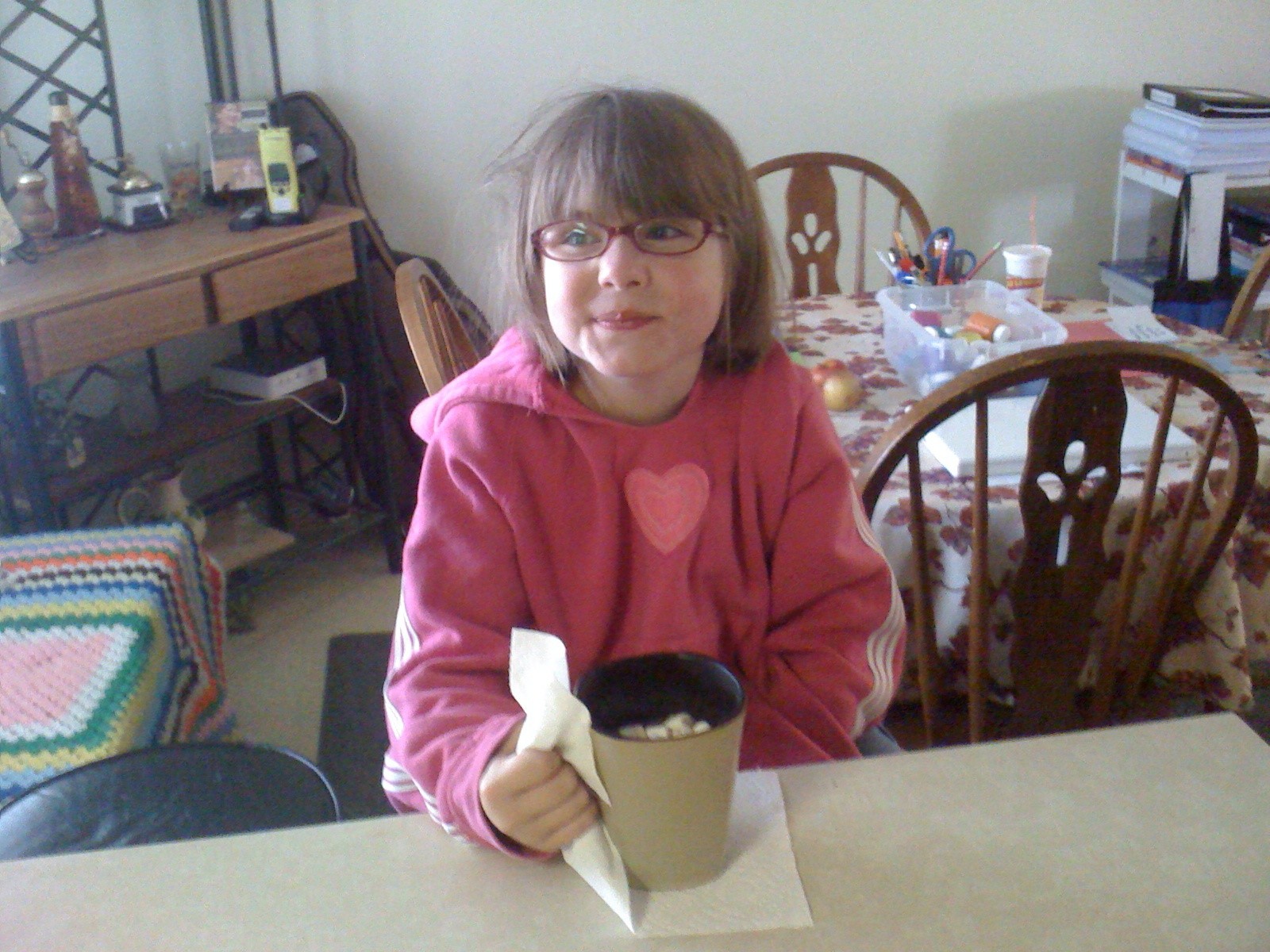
[155,138,204,211]
[576,653,749,893]
[1000,242,1052,314]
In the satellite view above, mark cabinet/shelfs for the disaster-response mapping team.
[1098,143,1270,340]
[0,188,407,591]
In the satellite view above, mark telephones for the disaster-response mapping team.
[256,121,326,225]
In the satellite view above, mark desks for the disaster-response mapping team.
[0,711,1270,952]
[774,290,1270,716]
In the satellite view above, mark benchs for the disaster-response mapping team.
[0,521,254,809]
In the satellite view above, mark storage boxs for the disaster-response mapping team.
[876,278,1070,399]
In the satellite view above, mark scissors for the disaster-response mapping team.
[923,227,976,286]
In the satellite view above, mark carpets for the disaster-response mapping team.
[316,633,399,821]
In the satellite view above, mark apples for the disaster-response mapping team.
[811,359,863,411]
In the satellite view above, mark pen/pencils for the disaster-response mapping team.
[874,230,949,285]
[965,241,1003,281]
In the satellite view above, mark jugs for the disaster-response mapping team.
[116,459,207,549]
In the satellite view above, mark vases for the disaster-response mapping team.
[113,460,206,544]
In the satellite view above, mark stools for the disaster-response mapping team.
[0,741,345,861]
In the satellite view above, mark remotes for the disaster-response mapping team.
[227,197,265,233]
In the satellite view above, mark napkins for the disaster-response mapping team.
[508,626,816,937]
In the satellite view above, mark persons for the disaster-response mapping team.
[382,99,912,869]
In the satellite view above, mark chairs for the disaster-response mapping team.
[394,257,482,396]
[748,153,937,299]
[853,340,1261,754]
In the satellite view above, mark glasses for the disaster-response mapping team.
[530,212,729,263]
